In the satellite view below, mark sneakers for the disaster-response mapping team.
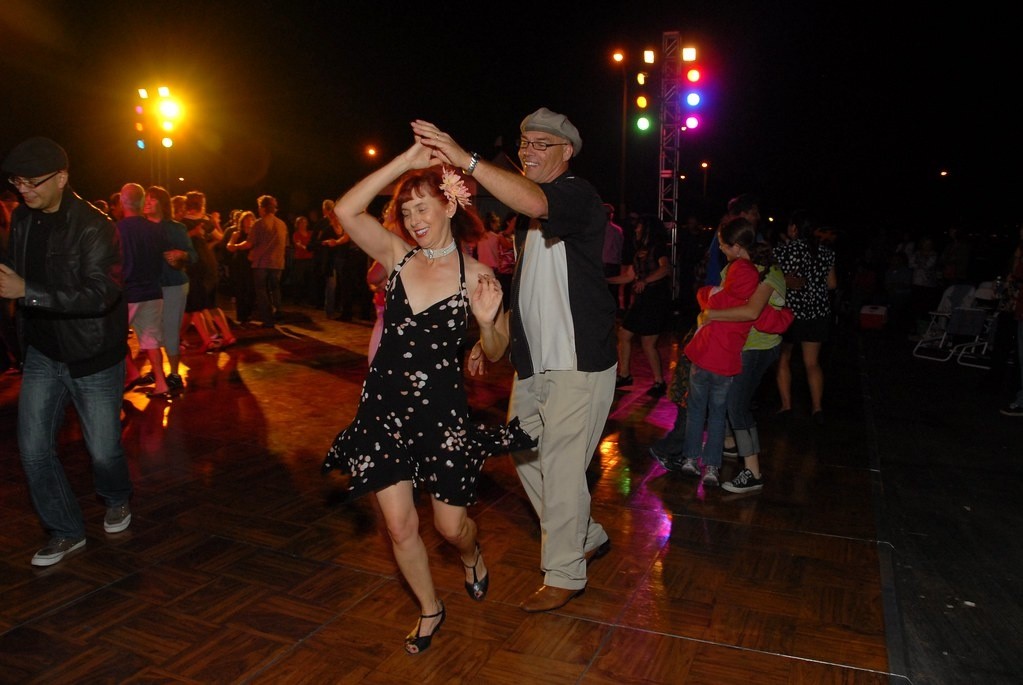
[722,444,738,457]
[721,468,764,493]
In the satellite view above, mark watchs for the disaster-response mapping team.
[462,151,482,176]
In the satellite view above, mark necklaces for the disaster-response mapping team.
[421,236,457,259]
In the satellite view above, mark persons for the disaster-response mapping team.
[0,183,519,398]
[0,137,130,565]
[321,135,539,654]
[600,196,1022,494]
[411,108,620,614]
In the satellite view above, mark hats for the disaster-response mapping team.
[0,134,69,177]
[520,106,582,156]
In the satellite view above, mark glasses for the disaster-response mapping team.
[8,171,59,189]
[520,139,568,150]
[720,262,730,290]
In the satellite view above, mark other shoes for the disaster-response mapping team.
[682,461,701,476]
[616,375,633,386]
[647,446,677,472]
[703,464,720,486]
[768,408,793,416]
[103,496,132,533]
[1000,402,1023,416]
[31,534,87,565]
[646,380,667,397]
[147,374,184,399]
[124,371,143,392]
[813,411,828,427]
[141,371,155,385]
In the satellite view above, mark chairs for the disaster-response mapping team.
[913,281,1011,369]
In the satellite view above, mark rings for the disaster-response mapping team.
[436,135,439,141]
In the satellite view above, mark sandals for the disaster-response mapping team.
[402,599,446,656]
[461,540,489,601]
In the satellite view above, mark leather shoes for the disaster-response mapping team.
[584,537,610,567]
[519,584,585,612]
